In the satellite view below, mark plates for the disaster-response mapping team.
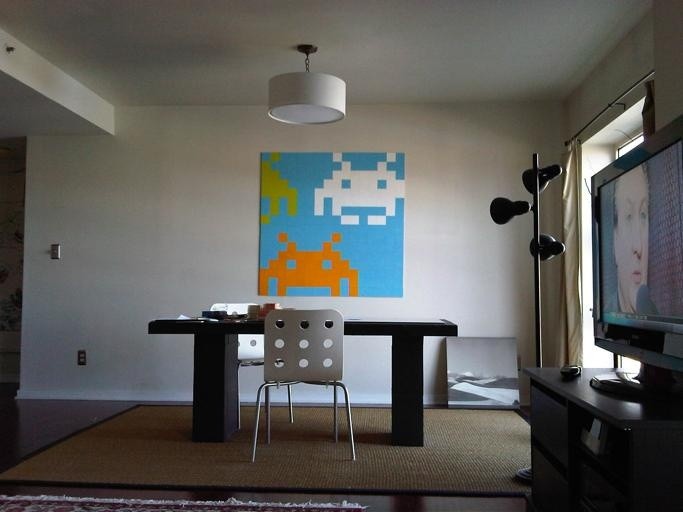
[245,318,257,320]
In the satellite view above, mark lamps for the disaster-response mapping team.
[490,153,565,365]
[268,44,347,123]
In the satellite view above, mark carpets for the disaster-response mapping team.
[0,399,532,498]
[0,494,370,512]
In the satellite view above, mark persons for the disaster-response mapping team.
[606,164,663,316]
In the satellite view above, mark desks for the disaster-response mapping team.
[523,367,683,512]
[149,312,458,447]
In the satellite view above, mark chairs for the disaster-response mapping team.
[250,309,361,460]
[210,303,296,433]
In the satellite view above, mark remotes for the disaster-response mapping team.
[560,365,581,380]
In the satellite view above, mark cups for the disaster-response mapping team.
[248,305,260,317]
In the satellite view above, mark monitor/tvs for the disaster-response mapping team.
[590,116,683,404]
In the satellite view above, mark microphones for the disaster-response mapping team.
[636,285,659,315]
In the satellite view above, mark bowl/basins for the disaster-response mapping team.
[202,310,227,320]
